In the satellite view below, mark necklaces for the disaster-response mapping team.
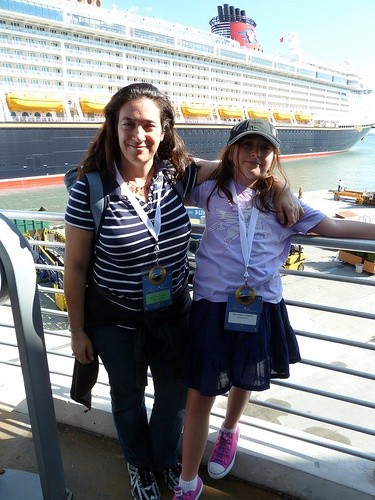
[122,172,152,195]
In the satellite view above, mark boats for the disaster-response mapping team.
[247,107,271,121]
[272,111,292,123]
[181,103,212,118]
[295,112,312,123]
[218,106,244,119]
[5,92,65,113]
[78,96,109,114]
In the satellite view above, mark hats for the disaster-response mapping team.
[228,119,280,149]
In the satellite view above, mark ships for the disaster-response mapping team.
[0,0,375,194]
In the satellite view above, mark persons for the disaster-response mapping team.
[338,179,341,192]
[64,83,224,500]
[177,119,375,500]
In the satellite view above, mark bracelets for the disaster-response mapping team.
[69,324,84,332]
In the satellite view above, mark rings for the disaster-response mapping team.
[72,353,75,356]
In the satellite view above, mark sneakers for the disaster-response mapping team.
[208,427,241,480]
[160,459,182,491]
[127,461,162,500]
[173,474,204,500]
